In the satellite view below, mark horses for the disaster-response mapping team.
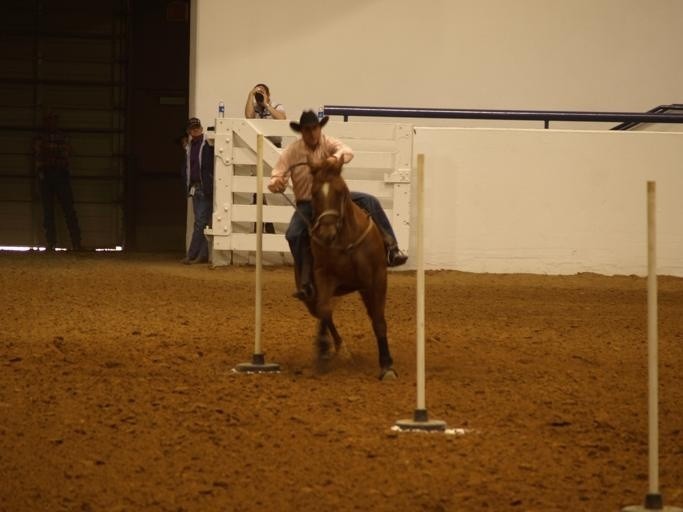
[301,153,399,381]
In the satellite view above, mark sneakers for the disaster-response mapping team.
[387,245,408,266]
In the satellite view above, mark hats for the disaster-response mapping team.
[187,118,201,128]
[290,111,329,131]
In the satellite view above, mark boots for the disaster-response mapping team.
[180,255,208,265]
[291,285,315,298]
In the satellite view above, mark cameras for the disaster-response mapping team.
[255,90,264,104]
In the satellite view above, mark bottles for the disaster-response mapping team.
[219,100,224,118]
[318,107,323,122]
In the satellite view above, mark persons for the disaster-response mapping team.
[185,116,214,265]
[267,108,409,300]
[31,111,89,253]
[245,83,287,148]
